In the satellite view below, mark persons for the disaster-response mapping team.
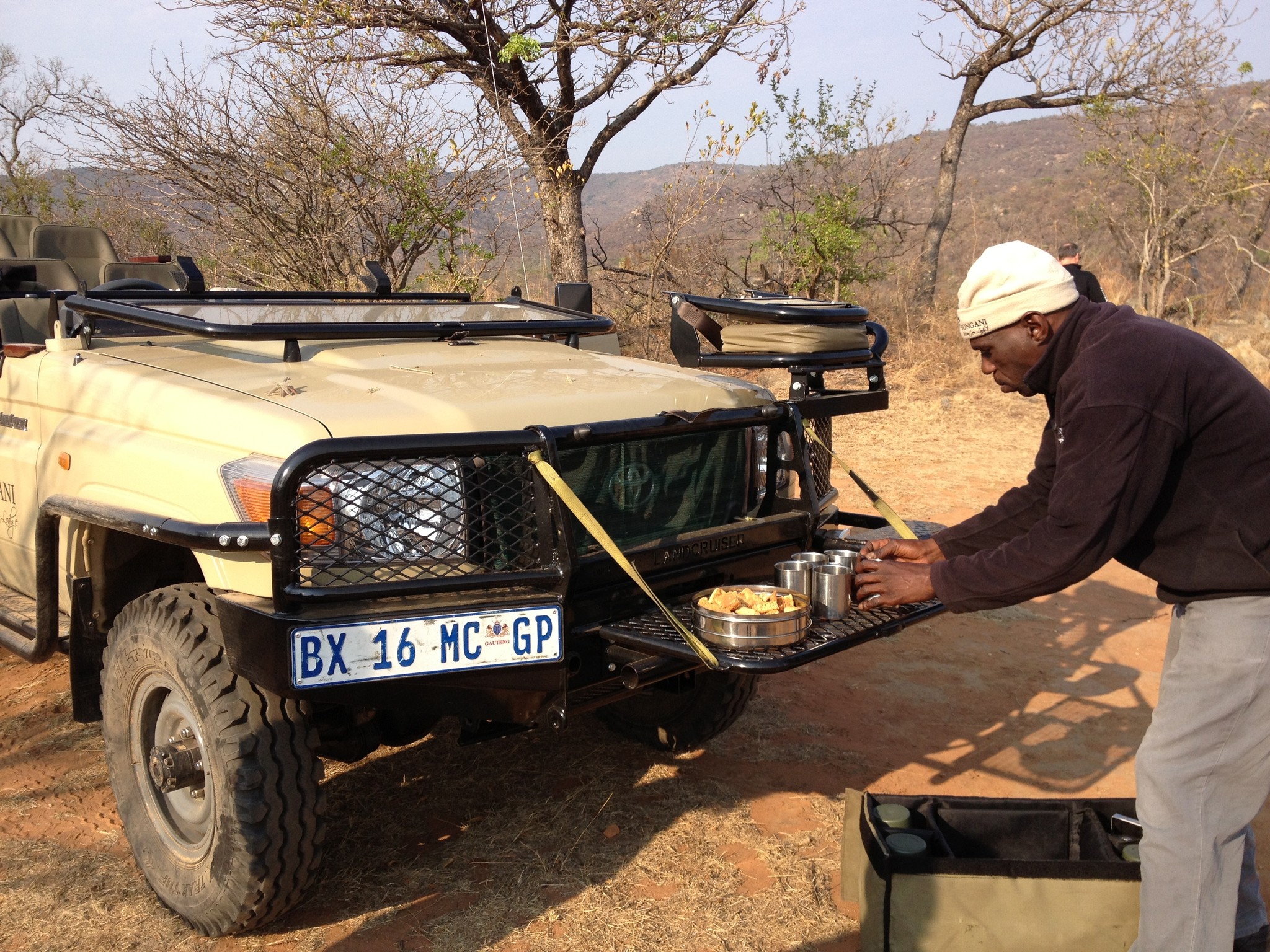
[1057,243,1108,303]
[853,241,1270,952]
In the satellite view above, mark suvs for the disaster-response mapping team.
[1,210,968,938]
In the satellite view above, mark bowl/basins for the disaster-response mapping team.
[691,585,813,649]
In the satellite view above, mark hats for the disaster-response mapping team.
[957,239,1080,339]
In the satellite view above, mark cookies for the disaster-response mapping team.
[698,588,800,616]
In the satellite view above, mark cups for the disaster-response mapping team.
[791,552,841,567]
[773,560,812,600]
[812,564,857,621]
[824,550,866,591]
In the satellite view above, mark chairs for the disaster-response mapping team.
[0,214,182,344]
